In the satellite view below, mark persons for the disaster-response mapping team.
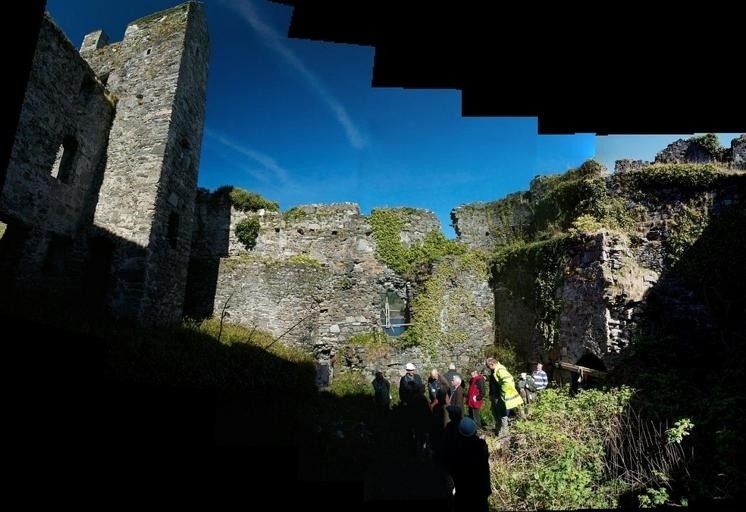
[372,357,549,512]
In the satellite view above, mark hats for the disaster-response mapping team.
[468,369,479,374]
[406,363,415,371]
[447,405,461,412]
[459,417,477,436]
[375,368,382,374]
